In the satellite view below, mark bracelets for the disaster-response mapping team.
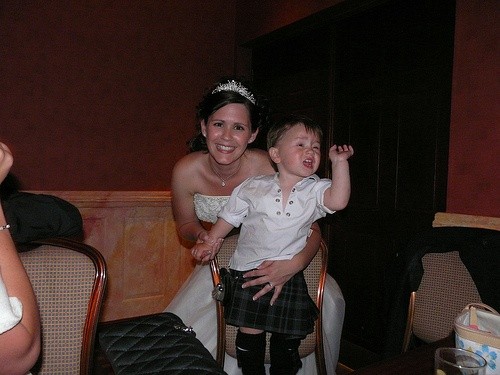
[0,224,11,231]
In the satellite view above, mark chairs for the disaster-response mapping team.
[12,237,108,375]
[404,252,485,352]
[209,228,327,375]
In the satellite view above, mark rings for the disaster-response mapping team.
[265,282,277,292]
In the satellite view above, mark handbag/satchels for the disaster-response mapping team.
[454,302,500,375]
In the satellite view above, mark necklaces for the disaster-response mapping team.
[199,152,248,197]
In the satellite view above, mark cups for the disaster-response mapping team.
[434,347,487,375]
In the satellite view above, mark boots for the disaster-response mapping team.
[235,328,302,375]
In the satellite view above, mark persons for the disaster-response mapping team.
[161,55,350,374]
[190,116,354,375]
[0,141,41,375]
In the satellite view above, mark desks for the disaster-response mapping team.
[338,331,462,375]
